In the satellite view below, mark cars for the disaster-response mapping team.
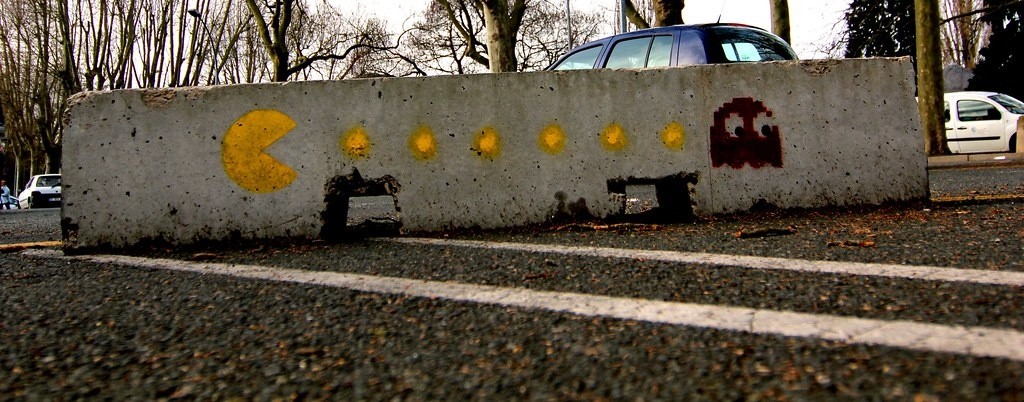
[914,89,1024,154]
[3,195,20,210]
[543,23,800,71]
[17,173,62,210]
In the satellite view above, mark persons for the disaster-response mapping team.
[0,179,11,210]
